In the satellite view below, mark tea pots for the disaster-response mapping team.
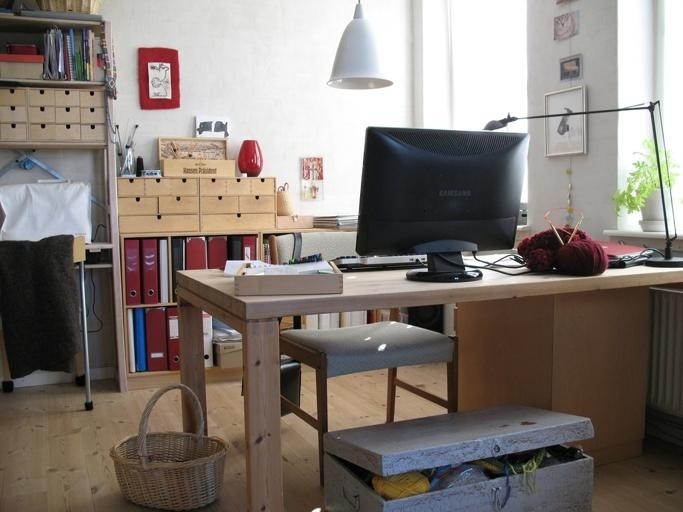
[43,25,63,80]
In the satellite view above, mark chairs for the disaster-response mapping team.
[267,221,461,489]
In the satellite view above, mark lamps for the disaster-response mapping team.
[325,1,395,91]
[484,101,683,268]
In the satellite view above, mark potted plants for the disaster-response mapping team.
[609,137,682,232]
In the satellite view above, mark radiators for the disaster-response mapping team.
[646,286,683,419]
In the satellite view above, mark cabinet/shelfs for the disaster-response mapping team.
[1,9,126,395]
[117,176,399,391]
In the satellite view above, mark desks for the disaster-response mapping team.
[175,254,683,511]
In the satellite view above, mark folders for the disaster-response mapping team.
[124,239,157,304]
[172,236,256,303]
[145,306,214,371]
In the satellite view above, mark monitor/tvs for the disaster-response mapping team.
[355,127,529,282]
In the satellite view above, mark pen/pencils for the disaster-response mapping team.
[283,252,323,265]
[513,255,525,265]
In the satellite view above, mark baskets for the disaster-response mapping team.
[109,382,229,511]
[277,190,293,216]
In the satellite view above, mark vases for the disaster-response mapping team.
[237,138,263,177]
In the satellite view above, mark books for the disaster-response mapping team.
[39,24,103,83]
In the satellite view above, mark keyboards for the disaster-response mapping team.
[334,254,427,272]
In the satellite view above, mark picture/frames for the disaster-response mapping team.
[543,83,589,160]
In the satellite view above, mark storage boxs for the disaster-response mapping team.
[211,340,244,370]
[1,51,47,81]
[321,405,594,512]
[157,136,237,178]
